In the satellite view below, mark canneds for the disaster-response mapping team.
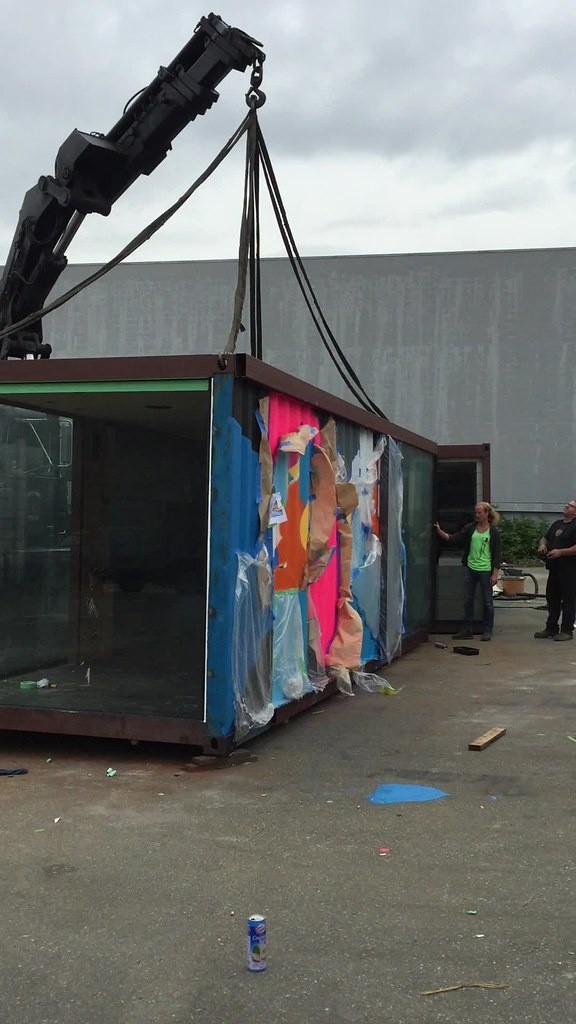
[245,912,267,972]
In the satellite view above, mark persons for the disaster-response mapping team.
[433,502,503,641]
[534,501,576,640]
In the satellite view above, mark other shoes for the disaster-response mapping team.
[535,626,560,638]
[555,632,572,641]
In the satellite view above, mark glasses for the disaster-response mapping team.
[566,503,576,509]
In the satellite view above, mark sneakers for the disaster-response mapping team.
[480,630,492,641]
[452,629,474,640]
